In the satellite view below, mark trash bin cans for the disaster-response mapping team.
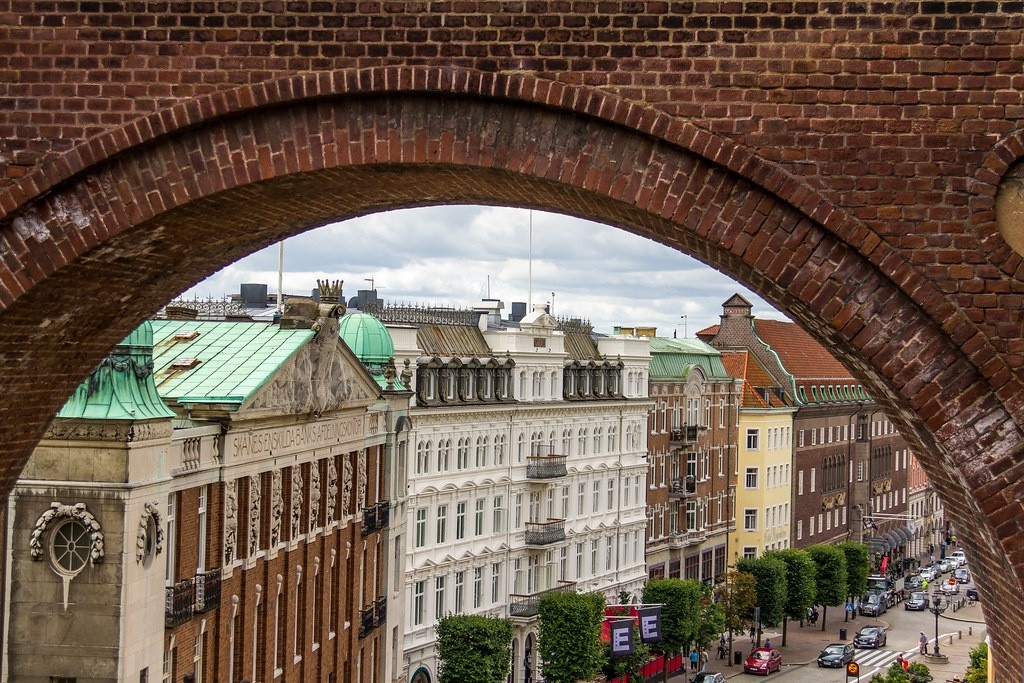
[734,650,742,665]
[839,628,847,640]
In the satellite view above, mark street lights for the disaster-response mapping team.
[923,581,951,663]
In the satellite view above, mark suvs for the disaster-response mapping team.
[853,625,887,649]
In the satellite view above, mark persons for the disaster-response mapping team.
[952,674,960,683]
[922,579,927,591]
[806,607,820,627]
[690,649,709,671]
[715,634,726,660]
[919,631,926,655]
[928,542,934,561]
[897,654,903,667]
[748,625,770,652]
[945,535,961,550]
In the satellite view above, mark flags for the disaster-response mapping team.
[638,607,661,643]
[610,620,634,655]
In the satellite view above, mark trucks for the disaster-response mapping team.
[865,574,905,607]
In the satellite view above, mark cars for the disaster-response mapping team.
[904,547,980,601]
[688,670,727,683]
[817,643,855,668]
[904,592,929,611]
[743,647,782,675]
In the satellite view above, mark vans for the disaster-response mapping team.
[858,593,886,616]
